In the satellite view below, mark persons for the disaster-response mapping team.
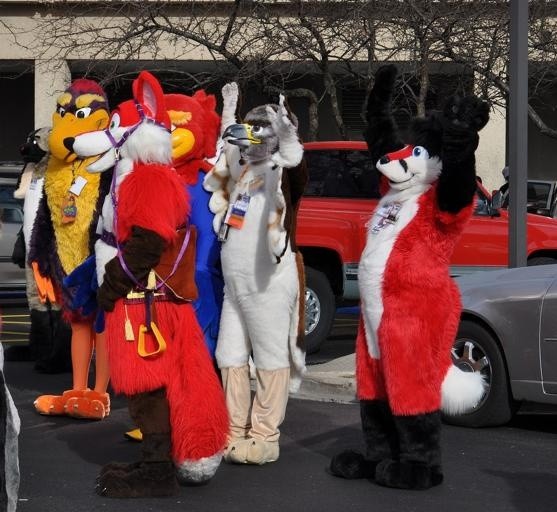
[500,166,513,210]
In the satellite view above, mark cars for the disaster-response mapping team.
[496,176,557,217]
[2,161,31,297]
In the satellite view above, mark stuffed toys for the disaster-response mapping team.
[13,70,309,499]
[331,64,489,491]
[11,71,309,483]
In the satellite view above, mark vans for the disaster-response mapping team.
[444,264,557,425]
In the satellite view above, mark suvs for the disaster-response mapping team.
[289,137,557,354]
